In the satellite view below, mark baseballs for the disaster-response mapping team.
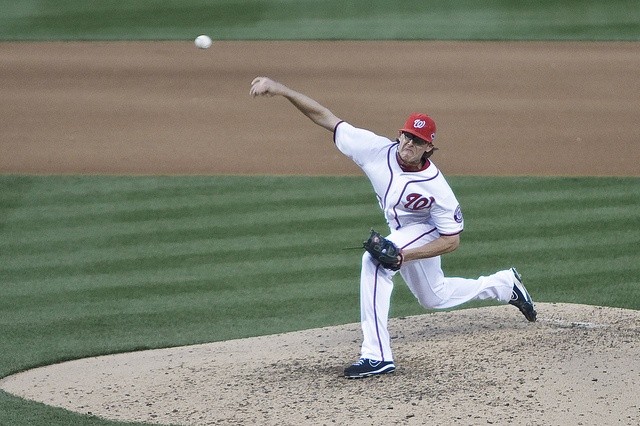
[195,34,211,49]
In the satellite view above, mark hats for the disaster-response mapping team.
[399,113,436,143]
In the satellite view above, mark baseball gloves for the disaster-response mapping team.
[343,228,403,271]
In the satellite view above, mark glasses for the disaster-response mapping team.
[405,133,427,145]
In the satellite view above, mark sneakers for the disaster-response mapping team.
[508,267,537,322]
[344,358,395,379]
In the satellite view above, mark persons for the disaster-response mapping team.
[249,76,537,379]
